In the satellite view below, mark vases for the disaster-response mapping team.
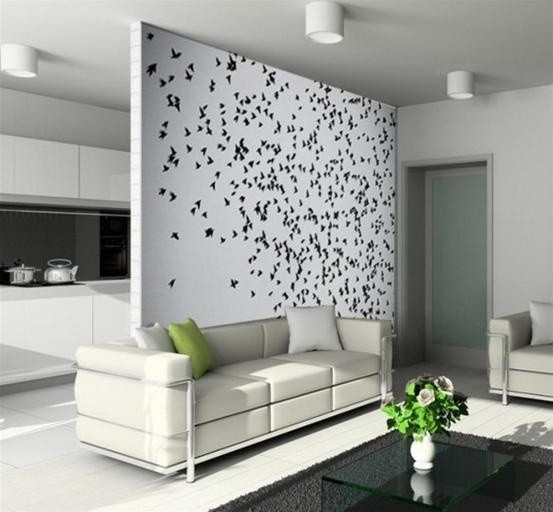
[410,469,436,506]
[410,430,435,469]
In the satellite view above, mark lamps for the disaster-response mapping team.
[448,71,475,100]
[305,2,345,44]
[1,44,38,79]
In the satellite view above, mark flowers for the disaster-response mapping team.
[381,372,469,443]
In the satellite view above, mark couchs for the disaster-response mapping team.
[75,318,396,483]
[486,312,553,405]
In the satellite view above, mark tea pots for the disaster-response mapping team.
[44,258,79,284]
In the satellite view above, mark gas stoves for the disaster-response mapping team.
[4,280,87,288]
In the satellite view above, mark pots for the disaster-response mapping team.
[0,264,17,285]
[4,262,42,284]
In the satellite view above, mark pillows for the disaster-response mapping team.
[529,301,553,346]
[133,318,218,380]
[283,304,344,354]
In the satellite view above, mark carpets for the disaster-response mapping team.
[209,428,553,512]
